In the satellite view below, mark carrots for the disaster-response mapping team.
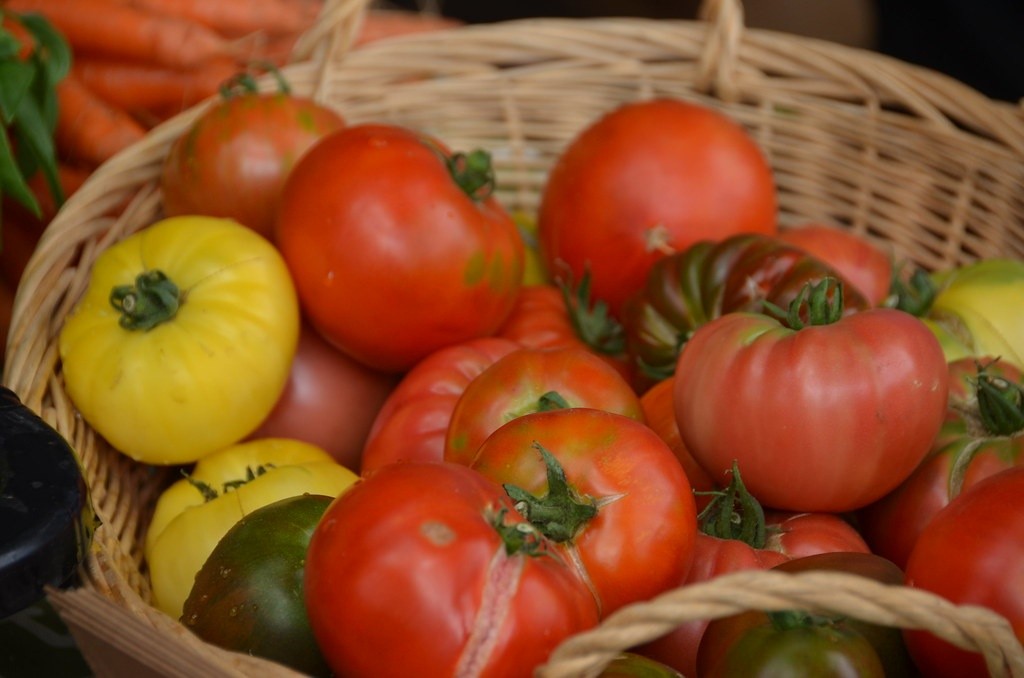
[0,1,460,378]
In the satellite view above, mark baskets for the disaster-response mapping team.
[0,2,1021,678]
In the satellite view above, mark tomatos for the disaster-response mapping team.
[54,63,1024,678]
